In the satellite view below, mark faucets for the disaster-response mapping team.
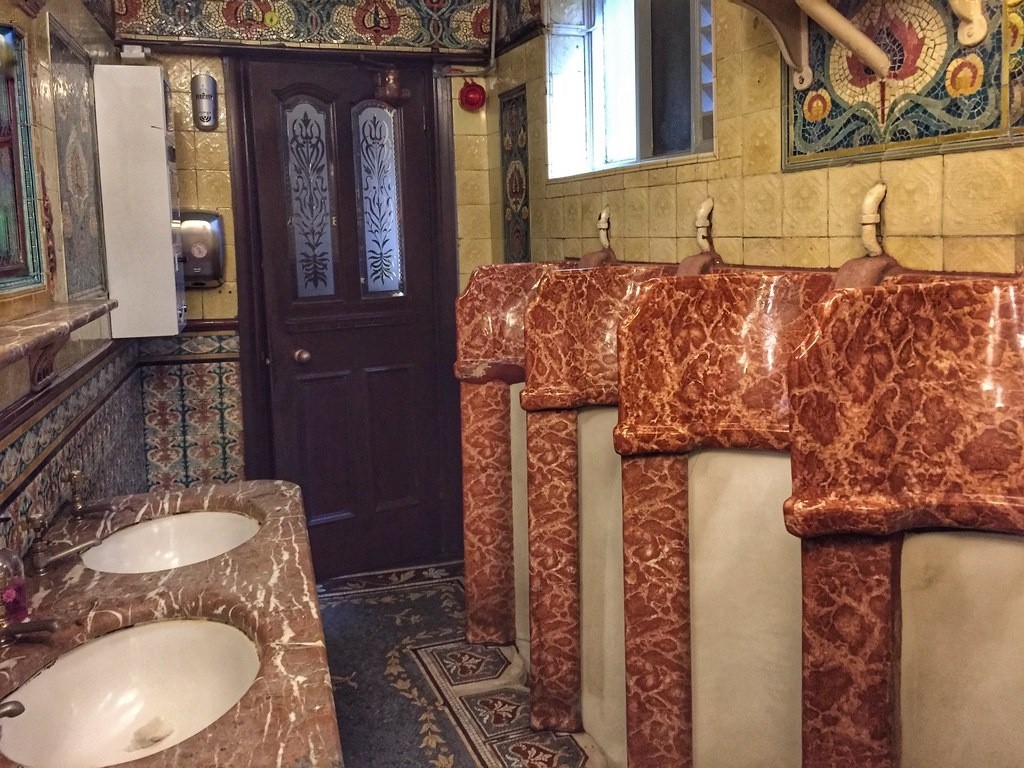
[0,599,62,649]
[29,513,103,576]
[68,469,119,524]
[0,700,26,720]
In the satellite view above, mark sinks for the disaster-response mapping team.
[0,617,262,768]
[79,510,263,575]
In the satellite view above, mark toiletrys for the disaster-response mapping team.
[0,516,29,625]
